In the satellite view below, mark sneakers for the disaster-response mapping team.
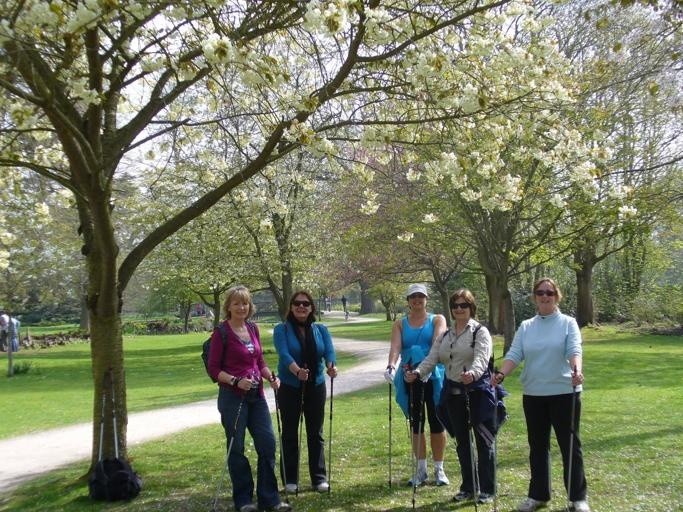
[239,503,257,512]
[477,492,495,503]
[408,472,428,486]
[573,500,590,511]
[285,484,298,494]
[518,497,548,510]
[435,473,449,486]
[314,482,330,492]
[453,490,474,500]
[270,502,291,512]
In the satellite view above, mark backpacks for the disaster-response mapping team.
[201,323,227,385]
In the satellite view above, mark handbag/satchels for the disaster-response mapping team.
[88,458,141,501]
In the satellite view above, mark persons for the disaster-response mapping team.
[274,291,338,491]
[0,311,10,352]
[8,315,21,352]
[406,288,497,504]
[342,295,348,312]
[384,283,448,486]
[490,277,590,512]
[208,286,290,512]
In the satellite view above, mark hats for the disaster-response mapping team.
[407,284,426,297]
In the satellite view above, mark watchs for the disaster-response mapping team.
[387,365,394,369]
[297,369,300,377]
[231,376,236,387]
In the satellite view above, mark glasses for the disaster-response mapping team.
[536,290,556,296]
[292,300,312,306]
[450,302,471,309]
